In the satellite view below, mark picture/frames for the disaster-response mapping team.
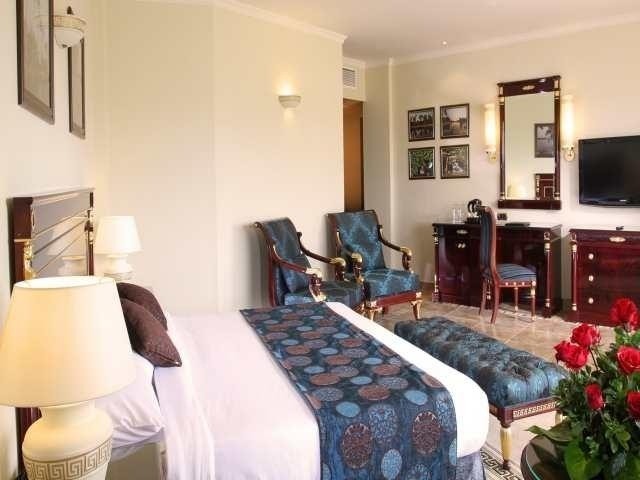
[535,123,555,158]
[67,6,86,138]
[408,146,436,179]
[439,103,470,138]
[440,143,470,179]
[16,1,55,125]
[407,106,435,141]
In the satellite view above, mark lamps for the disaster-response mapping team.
[484,104,497,162]
[92,215,141,280]
[54,15,87,48]
[46,216,90,277]
[1,275,139,480]
[277,85,303,115]
[563,95,576,160]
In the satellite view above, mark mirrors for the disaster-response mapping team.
[497,75,562,210]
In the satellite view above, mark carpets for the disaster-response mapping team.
[479,440,526,480]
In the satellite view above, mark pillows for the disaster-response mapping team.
[278,252,314,294]
[97,348,163,449]
[120,297,182,366]
[116,282,168,331]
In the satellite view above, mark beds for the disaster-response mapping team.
[12,187,490,480]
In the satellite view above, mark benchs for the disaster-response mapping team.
[396,315,573,469]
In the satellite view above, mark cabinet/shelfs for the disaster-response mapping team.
[565,226,639,329]
[431,220,563,319]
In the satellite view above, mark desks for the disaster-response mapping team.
[521,416,572,480]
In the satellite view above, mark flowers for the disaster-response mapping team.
[527,297,639,480]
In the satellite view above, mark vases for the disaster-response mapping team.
[584,410,637,462]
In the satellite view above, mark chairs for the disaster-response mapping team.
[252,216,365,318]
[478,206,537,323]
[328,208,422,320]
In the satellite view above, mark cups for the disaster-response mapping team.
[449,206,466,226]
[474,215,481,224]
[467,215,474,224]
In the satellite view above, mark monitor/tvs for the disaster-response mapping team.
[578,135,640,207]
